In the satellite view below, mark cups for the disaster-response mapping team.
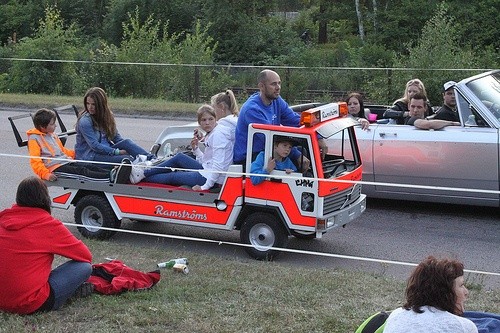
[367,114,377,123]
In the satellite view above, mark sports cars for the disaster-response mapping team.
[285,66,500,211]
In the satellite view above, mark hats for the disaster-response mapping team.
[442,81,458,92]
[276,134,302,147]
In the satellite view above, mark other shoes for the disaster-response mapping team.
[72,281,97,297]
[123,159,144,185]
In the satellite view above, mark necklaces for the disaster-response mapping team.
[447,106,457,112]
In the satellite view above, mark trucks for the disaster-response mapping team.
[6,99,368,263]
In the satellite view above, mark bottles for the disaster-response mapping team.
[157,257,189,269]
[464,114,476,127]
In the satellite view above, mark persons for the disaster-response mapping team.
[129,90,240,190]
[381,255,478,333]
[0,176,93,315]
[233,69,328,173]
[75,87,160,167]
[346,92,370,131]
[433,80,498,121]
[383,78,434,125]
[404,93,460,129]
[164,104,217,163]
[250,136,297,186]
[26,108,132,184]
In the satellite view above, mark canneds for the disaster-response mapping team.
[173,264,189,274]
